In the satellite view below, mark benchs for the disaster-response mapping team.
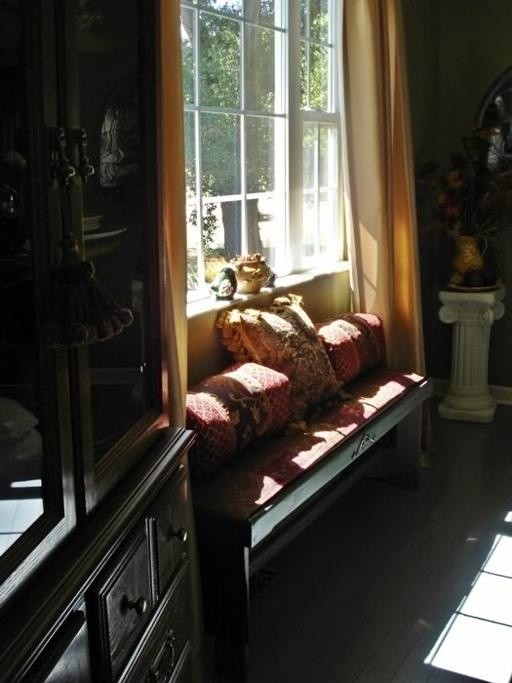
[191,368,437,683]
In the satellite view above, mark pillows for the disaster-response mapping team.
[312,312,388,384]
[186,362,292,482]
[215,294,349,439]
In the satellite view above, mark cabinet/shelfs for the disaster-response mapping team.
[2,0,208,683]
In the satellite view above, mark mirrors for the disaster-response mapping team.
[475,64,511,229]
